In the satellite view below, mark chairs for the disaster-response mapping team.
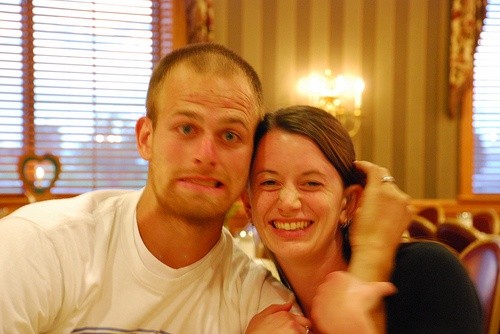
[403,205,500,334]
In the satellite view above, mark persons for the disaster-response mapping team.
[241,105,484,334]
[0,42,410,334]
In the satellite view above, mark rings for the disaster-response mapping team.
[381,176,394,182]
[305,325,310,334]
